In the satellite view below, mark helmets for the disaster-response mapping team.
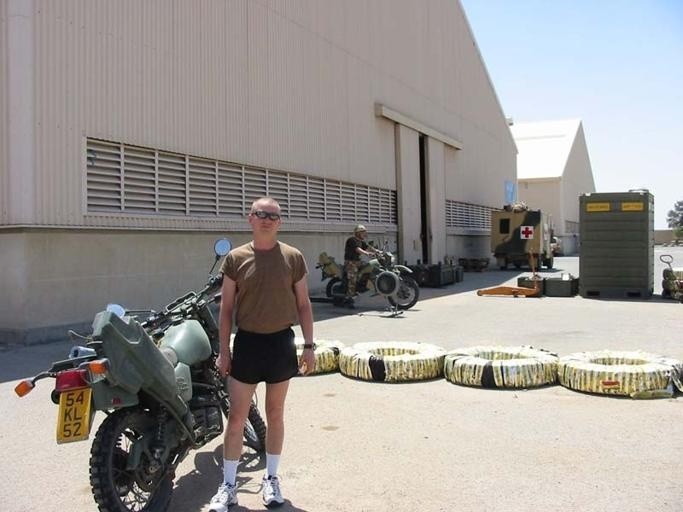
[354,223,367,232]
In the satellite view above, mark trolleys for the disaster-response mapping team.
[659,254,682,303]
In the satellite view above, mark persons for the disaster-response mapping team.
[206,197,316,512]
[342,224,383,307]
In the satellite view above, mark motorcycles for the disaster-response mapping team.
[13,237,266,512]
[315,240,419,318]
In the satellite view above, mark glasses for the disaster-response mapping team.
[251,211,280,220]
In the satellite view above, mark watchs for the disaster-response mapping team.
[304,343,316,350]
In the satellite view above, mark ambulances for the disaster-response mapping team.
[490,200,553,270]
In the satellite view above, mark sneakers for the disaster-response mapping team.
[263,474,284,507]
[208,482,238,512]
